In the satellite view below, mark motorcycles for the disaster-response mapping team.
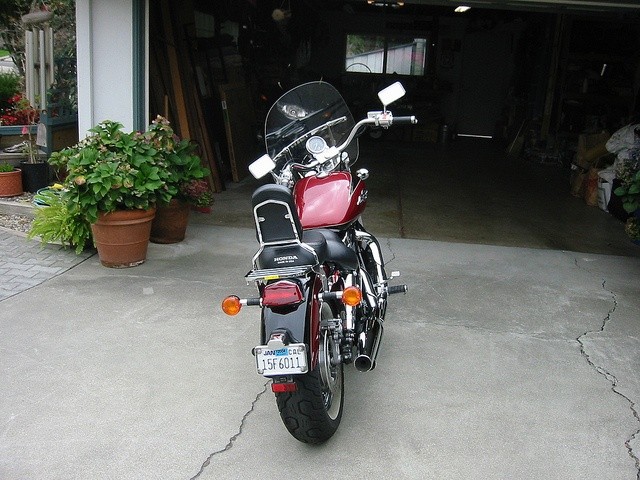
[222,81,417,445]
